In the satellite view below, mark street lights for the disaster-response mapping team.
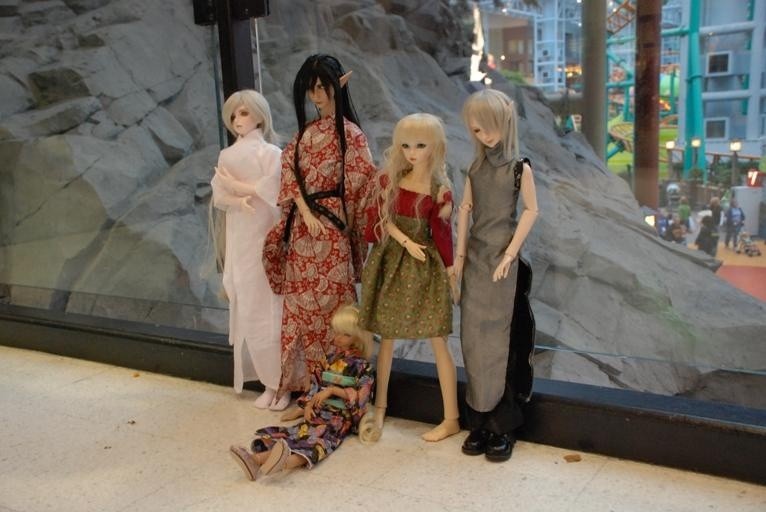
[665,141,675,179]
[691,139,700,167]
[730,142,741,187]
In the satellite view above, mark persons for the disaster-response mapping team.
[449,89,537,460]
[724,199,745,249]
[660,215,687,247]
[212,89,292,411]
[695,216,718,255]
[678,196,692,232]
[710,197,722,226]
[357,112,461,443]
[230,307,375,481]
[211,54,538,481]
[262,55,378,411]
